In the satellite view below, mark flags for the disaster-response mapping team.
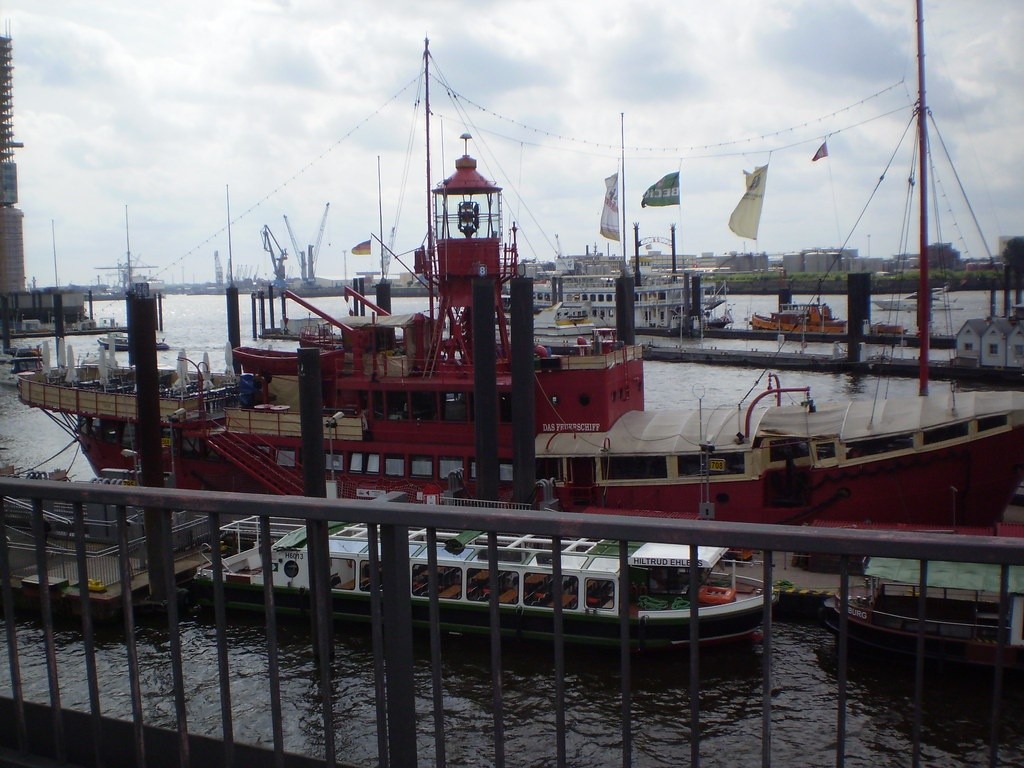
[812,142,829,162]
[726,163,769,240]
[351,240,372,255]
[641,171,680,208]
[600,172,621,241]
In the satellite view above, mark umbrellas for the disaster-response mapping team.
[41,335,235,399]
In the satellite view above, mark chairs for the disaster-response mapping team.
[361,568,612,610]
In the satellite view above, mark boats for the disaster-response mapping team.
[824,558,1024,653]
[1,133,1024,526]
[752,302,908,335]
[872,288,964,310]
[194,515,776,657]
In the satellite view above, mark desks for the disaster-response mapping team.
[472,570,490,586]
[498,589,519,603]
[421,567,445,576]
[439,584,462,598]
[525,574,548,585]
[546,594,576,608]
[339,576,367,590]
